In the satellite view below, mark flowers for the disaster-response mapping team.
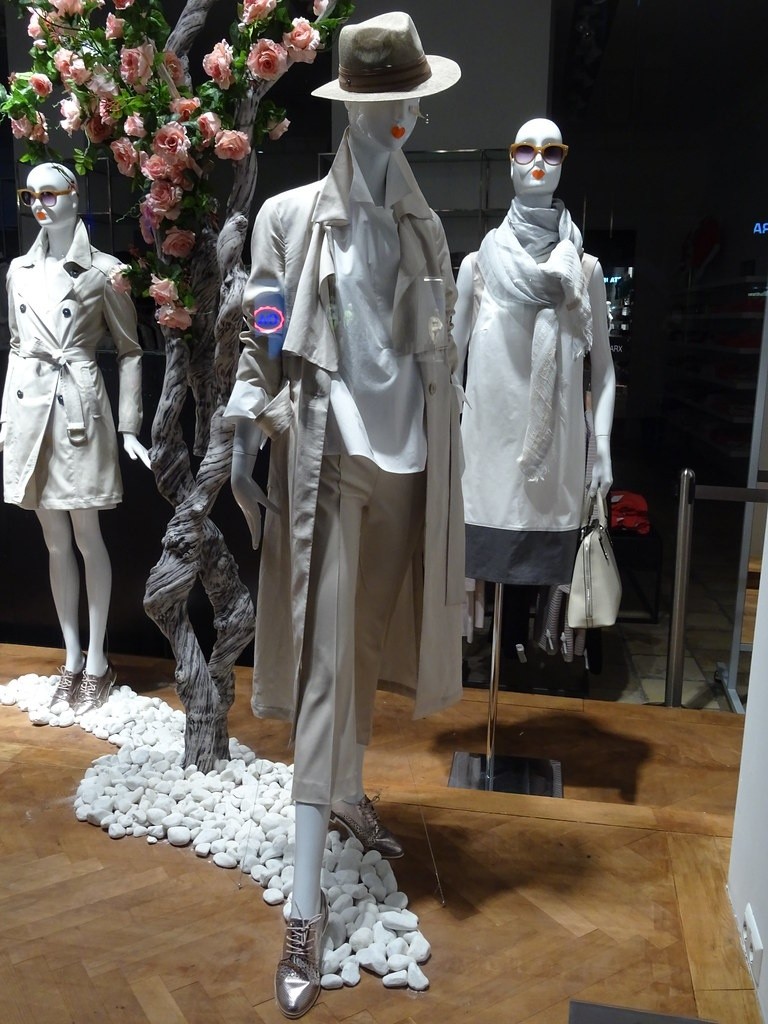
[0,0,356,348]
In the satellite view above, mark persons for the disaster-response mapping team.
[222,11,460,1021]
[449,116,616,585]
[1,162,152,717]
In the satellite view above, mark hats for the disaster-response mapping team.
[310,11,463,103]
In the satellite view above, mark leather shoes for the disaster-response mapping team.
[72,658,117,717]
[49,652,87,715]
[329,794,406,860]
[272,888,330,1019]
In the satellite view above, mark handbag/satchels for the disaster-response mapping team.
[567,487,623,628]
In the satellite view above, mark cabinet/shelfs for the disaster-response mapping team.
[317,148,509,271]
[659,275,768,487]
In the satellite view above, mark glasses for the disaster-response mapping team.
[509,141,568,166]
[16,189,73,208]
